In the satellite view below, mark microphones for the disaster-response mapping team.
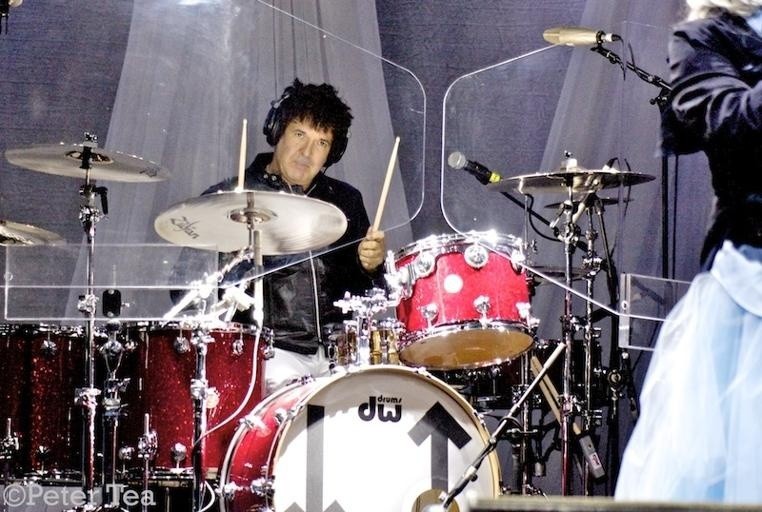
[543,27,622,46]
[448,151,504,183]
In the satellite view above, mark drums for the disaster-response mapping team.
[0,323,110,484]
[443,339,602,411]
[383,231,547,373]
[106,318,274,489]
[215,365,502,512]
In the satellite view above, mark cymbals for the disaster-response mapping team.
[154,189,347,255]
[543,197,634,209]
[0,220,68,247]
[536,265,596,288]
[6,141,169,183]
[485,160,657,191]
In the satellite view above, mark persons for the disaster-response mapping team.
[600,0,762,507]
[172,75,387,397]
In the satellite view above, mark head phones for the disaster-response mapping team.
[264,90,348,168]
